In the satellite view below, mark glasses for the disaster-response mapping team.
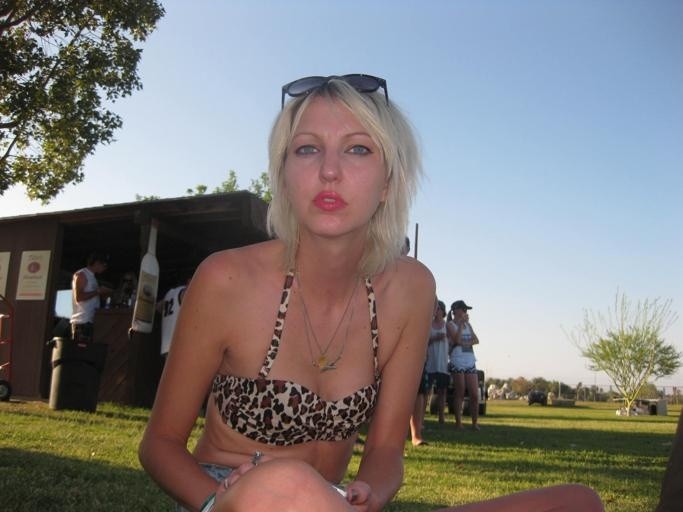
[281,73,390,110]
[459,307,467,313]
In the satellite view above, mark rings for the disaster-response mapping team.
[251,449,261,463]
[224,479,227,489]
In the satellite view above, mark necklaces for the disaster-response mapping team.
[297,271,358,372]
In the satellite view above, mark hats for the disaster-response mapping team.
[452,301,472,309]
[438,301,446,317]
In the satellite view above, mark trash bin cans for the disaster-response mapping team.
[45,336,108,415]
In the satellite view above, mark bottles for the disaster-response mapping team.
[130,224,161,336]
[105,296,112,312]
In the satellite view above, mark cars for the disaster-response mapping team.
[528,391,547,406]
[430,370,486,416]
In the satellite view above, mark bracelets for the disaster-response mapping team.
[196,496,216,512]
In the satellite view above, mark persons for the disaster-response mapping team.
[426,301,449,429]
[446,300,482,432]
[115,269,136,305]
[72,248,116,344]
[156,270,196,371]
[400,236,432,449]
[136,70,604,512]
